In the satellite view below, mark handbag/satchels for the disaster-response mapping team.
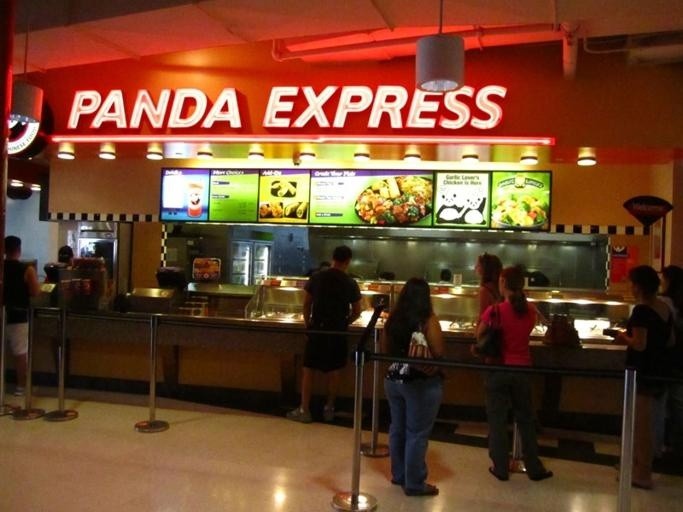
[476,326,504,357]
[399,330,442,379]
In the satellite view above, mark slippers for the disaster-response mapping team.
[617,473,655,492]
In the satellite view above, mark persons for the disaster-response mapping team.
[650,263,682,476]
[1,234,42,397]
[378,276,450,497]
[305,261,331,277]
[469,264,554,483]
[438,267,452,283]
[44,243,76,312]
[287,243,361,426]
[474,251,503,327]
[611,263,671,493]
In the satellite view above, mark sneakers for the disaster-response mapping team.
[489,467,509,482]
[528,471,553,482]
[390,476,439,496]
[286,407,311,423]
[14,387,25,396]
[324,408,335,422]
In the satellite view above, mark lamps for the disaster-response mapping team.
[52,143,599,167]
[414,2,463,95]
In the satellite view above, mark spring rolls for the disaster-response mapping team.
[285,202,299,217]
[296,202,307,218]
[271,180,296,196]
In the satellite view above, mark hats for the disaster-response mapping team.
[58,246,74,262]
